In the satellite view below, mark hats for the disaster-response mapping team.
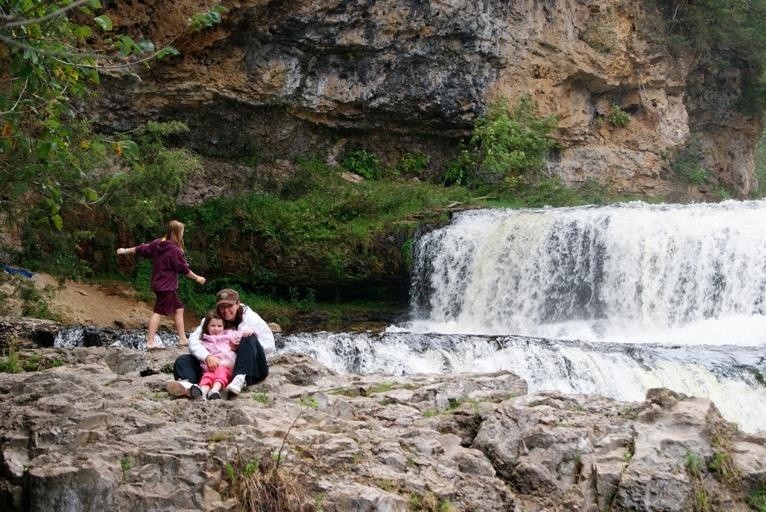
[216,288,238,306]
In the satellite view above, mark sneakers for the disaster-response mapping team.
[167,378,246,399]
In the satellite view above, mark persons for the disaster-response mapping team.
[165,288,277,399]
[115,220,208,348]
[189,312,246,400]
